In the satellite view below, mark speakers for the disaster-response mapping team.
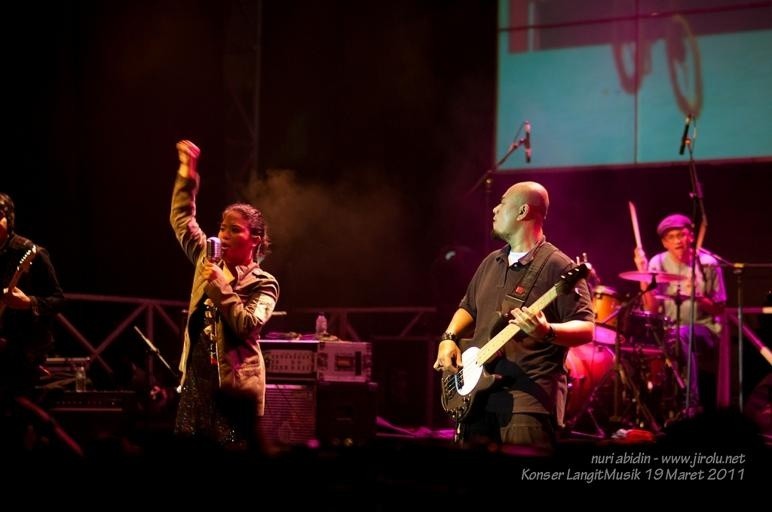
[259,382,376,454]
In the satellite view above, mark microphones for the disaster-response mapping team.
[679,113,691,155]
[206,237,221,264]
[523,120,531,163]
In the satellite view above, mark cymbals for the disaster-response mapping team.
[619,271,688,282]
[654,294,703,302]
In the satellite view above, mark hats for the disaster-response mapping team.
[656,212,694,237]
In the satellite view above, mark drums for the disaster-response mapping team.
[557,342,615,428]
[590,285,629,346]
[609,346,671,429]
[630,310,672,345]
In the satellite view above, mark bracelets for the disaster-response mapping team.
[544,324,556,345]
[440,331,457,341]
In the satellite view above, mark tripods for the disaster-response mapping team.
[565,302,661,438]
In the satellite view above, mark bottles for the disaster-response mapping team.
[74,365,86,394]
[315,310,328,336]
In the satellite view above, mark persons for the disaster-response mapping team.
[1,194,72,397]
[433,180,597,459]
[634,213,729,413]
[170,140,279,450]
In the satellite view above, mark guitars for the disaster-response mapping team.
[0,247,36,315]
[439,253,593,422]
